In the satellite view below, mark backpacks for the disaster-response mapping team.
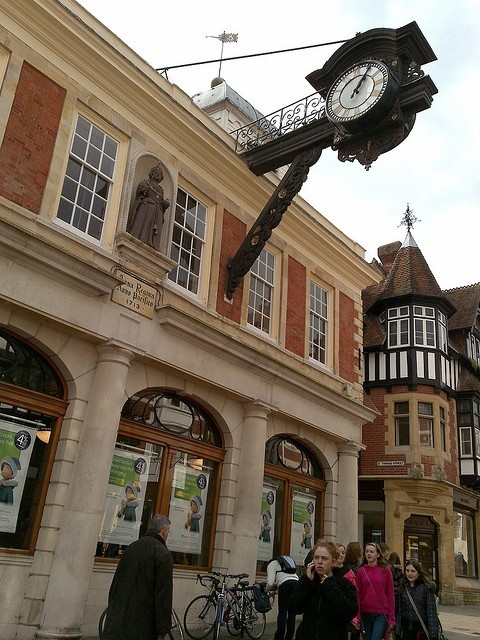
[276,554,296,573]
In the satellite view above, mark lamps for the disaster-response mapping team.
[188,459,204,471]
[36,430,52,444]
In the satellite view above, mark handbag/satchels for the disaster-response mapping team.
[438,628,449,640]
[385,627,396,640]
[253,582,272,613]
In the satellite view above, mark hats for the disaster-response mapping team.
[263,511,272,523]
[125,480,140,499]
[304,519,312,531]
[0,456,21,478]
[190,496,203,512]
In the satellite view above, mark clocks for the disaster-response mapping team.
[324,60,389,122]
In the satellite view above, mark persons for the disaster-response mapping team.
[332,542,358,626]
[386,551,404,640]
[127,165,171,253]
[263,559,299,640]
[395,559,440,639]
[185,495,203,533]
[0,456,21,505]
[342,542,363,572]
[259,511,272,542]
[101,513,174,639]
[300,519,313,549]
[116,480,141,521]
[378,543,390,560]
[356,542,395,640]
[292,537,359,640]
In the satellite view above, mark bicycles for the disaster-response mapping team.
[184,574,255,639]
[207,571,266,640]
[99,607,184,640]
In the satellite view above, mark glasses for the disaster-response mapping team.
[367,542,376,546]
